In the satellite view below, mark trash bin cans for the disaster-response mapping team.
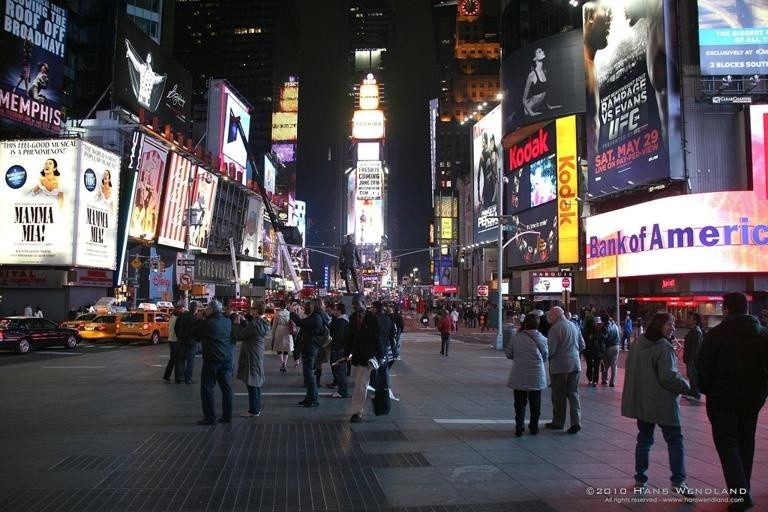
[503,322,517,348]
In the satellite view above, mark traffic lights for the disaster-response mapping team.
[257,245,261,254]
[536,238,544,251]
[458,250,466,264]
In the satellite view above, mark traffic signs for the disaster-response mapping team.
[500,223,519,233]
[177,260,195,266]
[187,249,201,255]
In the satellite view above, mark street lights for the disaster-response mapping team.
[494,163,520,349]
[409,268,421,286]
[183,175,211,314]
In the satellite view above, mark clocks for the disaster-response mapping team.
[459,0,481,15]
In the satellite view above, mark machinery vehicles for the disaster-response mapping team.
[226,237,250,311]
[232,114,338,316]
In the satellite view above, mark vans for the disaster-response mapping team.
[192,295,217,308]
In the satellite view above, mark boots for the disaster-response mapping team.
[316,368,323,388]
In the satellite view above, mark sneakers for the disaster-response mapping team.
[217,418,231,423]
[671,482,696,499]
[588,381,615,387]
[240,409,262,417]
[514,423,585,438]
[728,492,753,512]
[196,419,216,425]
[326,382,375,398]
[350,414,363,422]
[635,481,649,494]
[686,396,700,401]
[163,378,193,384]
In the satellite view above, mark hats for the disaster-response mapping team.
[532,48,546,62]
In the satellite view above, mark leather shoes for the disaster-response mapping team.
[298,400,319,407]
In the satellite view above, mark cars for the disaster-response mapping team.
[61,299,180,347]
[0,316,80,352]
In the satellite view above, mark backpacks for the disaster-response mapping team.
[310,313,333,349]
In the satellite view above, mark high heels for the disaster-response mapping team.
[280,362,287,372]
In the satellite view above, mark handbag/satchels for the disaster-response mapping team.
[372,355,391,417]
[289,320,298,336]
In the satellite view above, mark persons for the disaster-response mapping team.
[339,235,362,293]
[441,267,451,286]
[11,302,95,321]
[125,41,167,111]
[27,62,50,105]
[27,159,64,207]
[94,170,113,215]
[13,30,33,98]
